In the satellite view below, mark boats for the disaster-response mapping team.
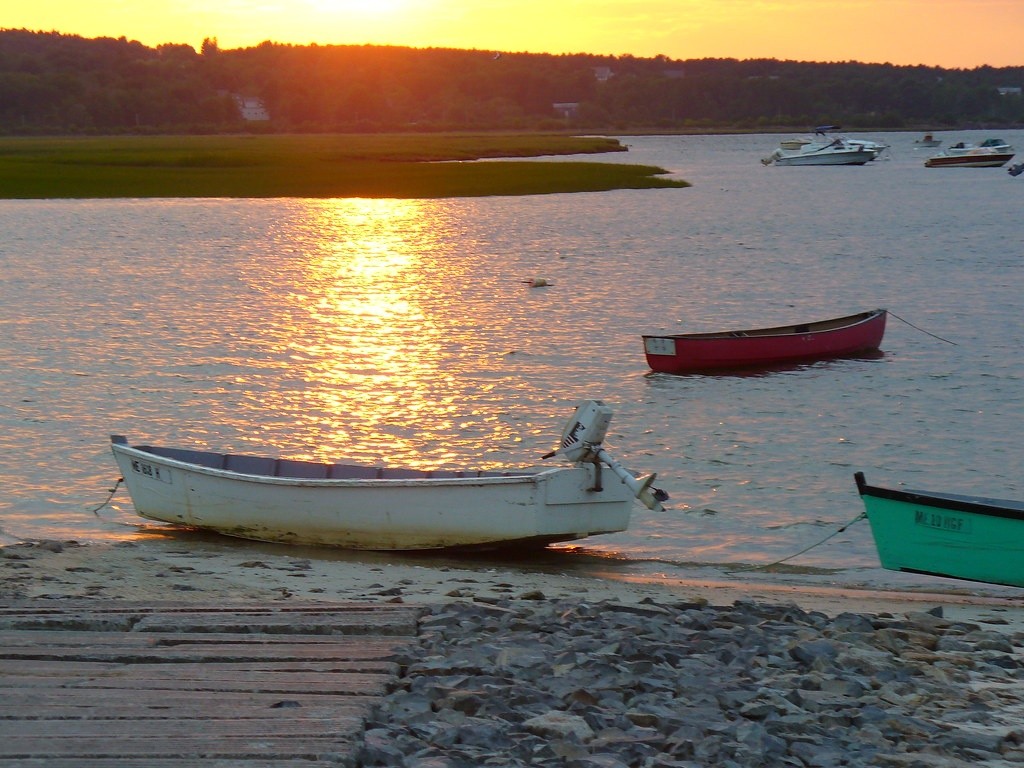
[106,396,671,553]
[942,138,1013,155]
[811,124,891,162]
[779,137,810,151]
[924,146,1016,167]
[758,134,876,166]
[852,470,1024,591]
[912,131,944,147]
[641,308,890,373]
